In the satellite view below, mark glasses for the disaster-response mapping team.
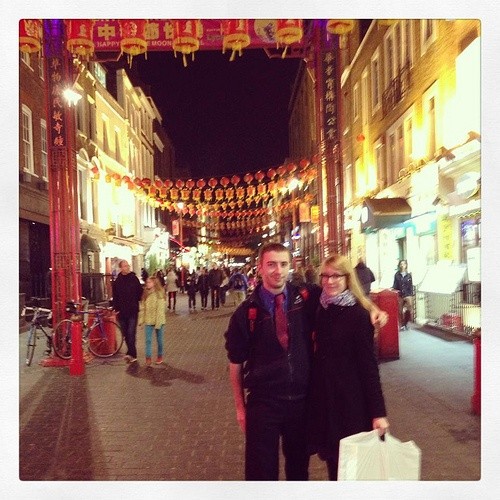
[319,273,347,281]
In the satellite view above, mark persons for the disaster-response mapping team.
[112,259,144,363]
[137,276,167,367]
[353,259,375,295]
[393,259,413,331]
[139,262,258,312]
[224,243,389,481]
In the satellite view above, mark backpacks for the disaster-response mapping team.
[234,278,243,288]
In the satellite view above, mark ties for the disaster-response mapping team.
[274,294,289,353]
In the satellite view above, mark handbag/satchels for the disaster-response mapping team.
[336,427,422,481]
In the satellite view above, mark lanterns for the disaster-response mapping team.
[19,19,356,59]
[92,159,316,250]
[356,135,365,142]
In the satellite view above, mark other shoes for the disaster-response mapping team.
[144,358,152,367]
[124,356,138,363]
[155,356,163,365]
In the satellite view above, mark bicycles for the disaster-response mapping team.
[19,304,53,367]
[50,295,125,360]
